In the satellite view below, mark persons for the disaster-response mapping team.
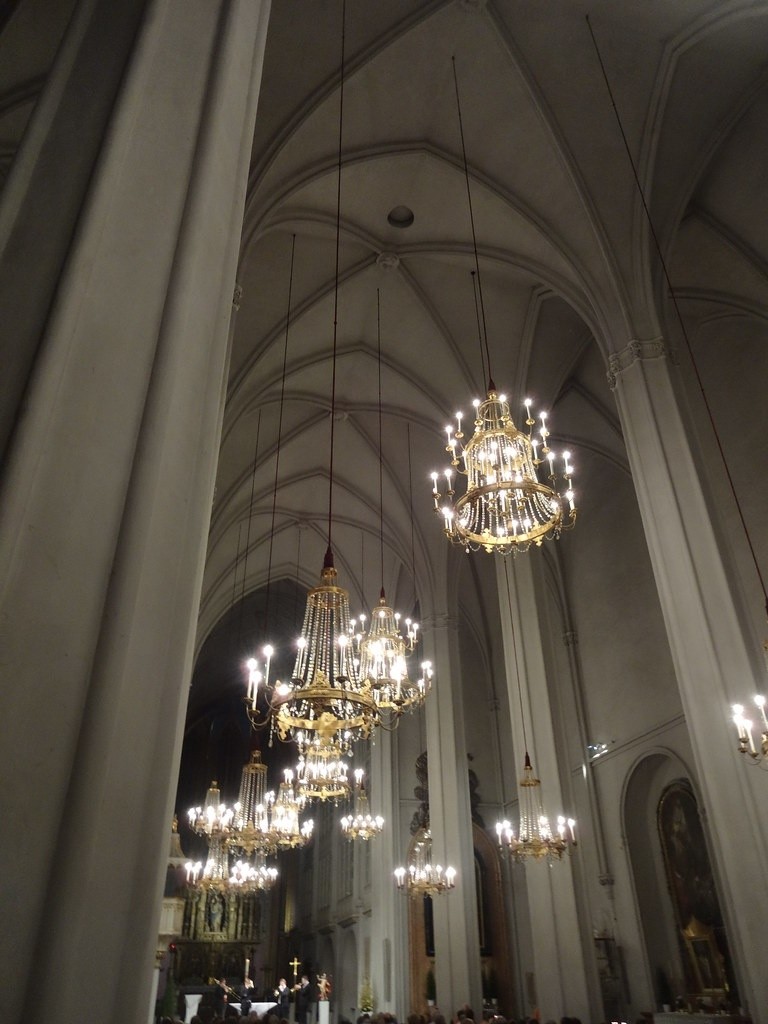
[274,978,290,1019]
[357,1004,582,1024]
[240,976,253,1016]
[190,1000,289,1024]
[214,978,228,1021]
[295,976,312,1024]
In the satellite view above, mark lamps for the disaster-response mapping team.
[170,1,768,909]
